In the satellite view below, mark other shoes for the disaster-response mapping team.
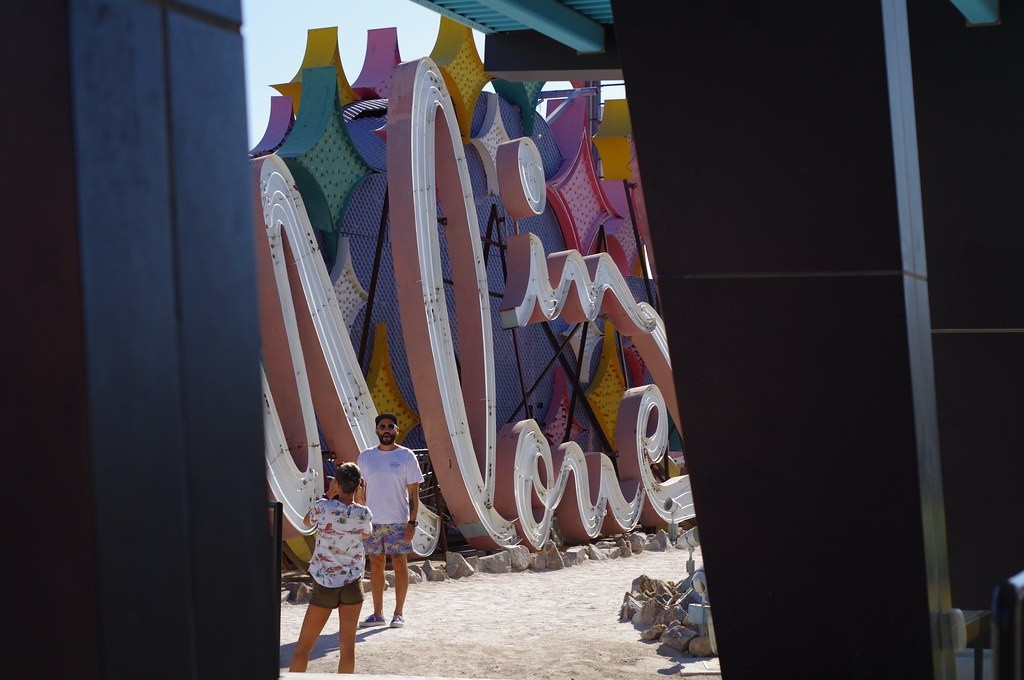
[359,614,385,627]
[390,615,405,628]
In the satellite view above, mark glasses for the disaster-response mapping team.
[377,424,398,430]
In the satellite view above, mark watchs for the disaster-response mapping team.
[408,520,419,527]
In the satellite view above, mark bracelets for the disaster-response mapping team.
[322,493,330,500]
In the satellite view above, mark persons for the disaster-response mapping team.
[289,462,374,674]
[357,413,424,628]
